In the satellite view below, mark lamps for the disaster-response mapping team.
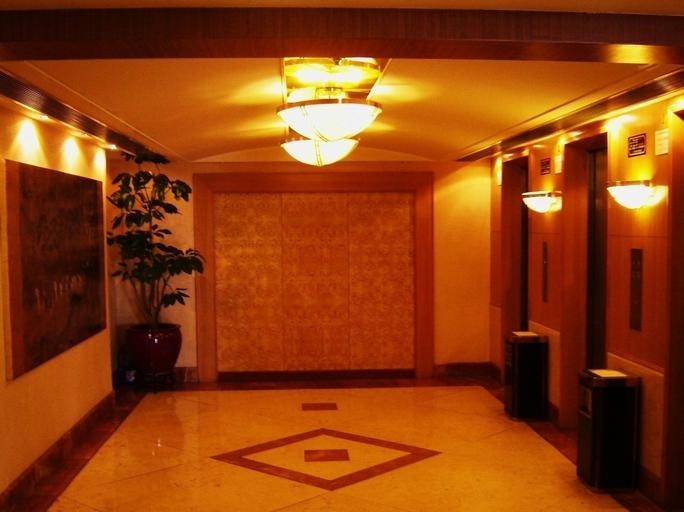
[604,179,653,211]
[274,81,383,169]
[520,190,563,214]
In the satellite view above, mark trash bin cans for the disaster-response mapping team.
[501,331,548,419]
[576,369,641,489]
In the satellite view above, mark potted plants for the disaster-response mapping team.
[106,139,208,385]
